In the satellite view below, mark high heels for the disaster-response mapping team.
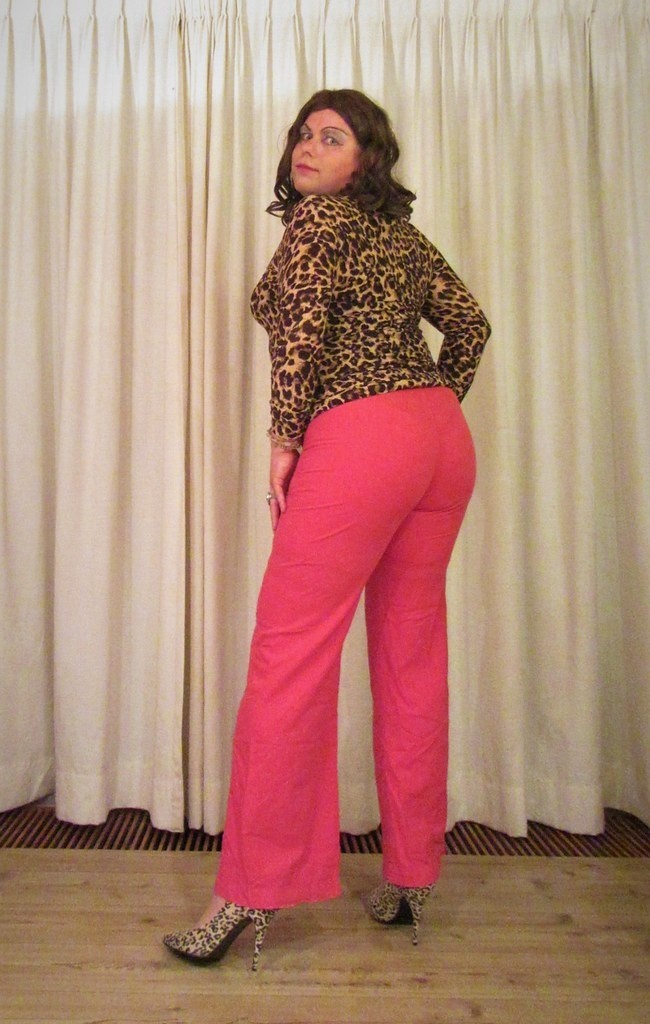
[162,901,278,971]
[364,880,433,945]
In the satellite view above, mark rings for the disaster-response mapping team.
[266,492,277,507]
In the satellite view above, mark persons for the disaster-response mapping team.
[164,85,492,971]
